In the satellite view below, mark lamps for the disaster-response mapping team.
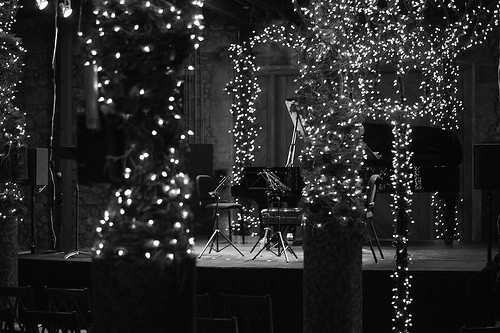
[35,0,49,10]
[58,0,72,18]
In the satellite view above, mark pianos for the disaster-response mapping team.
[232,99,463,246]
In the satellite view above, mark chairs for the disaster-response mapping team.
[0,285,95,333]
[362,173,385,265]
[196,291,275,333]
[195,174,244,258]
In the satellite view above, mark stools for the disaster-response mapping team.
[261,208,302,262]
